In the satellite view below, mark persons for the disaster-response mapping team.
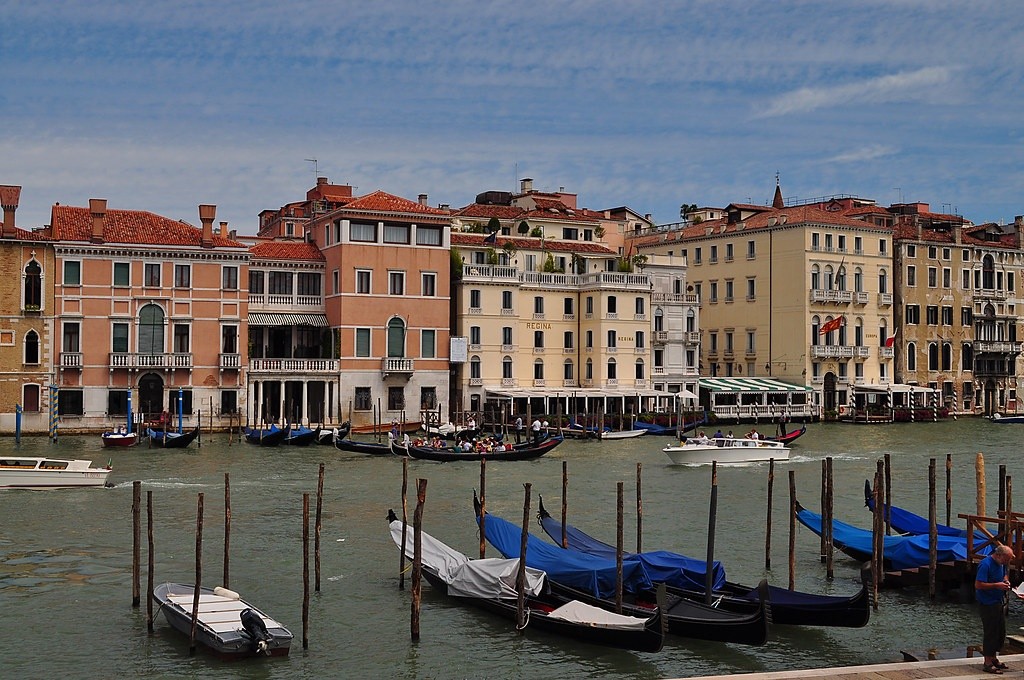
[725,431,735,446]
[542,419,549,427]
[392,423,398,444]
[530,417,541,448]
[713,430,724,442]
[514,413,522,444]
[456,436,513,453]
[974,546,1013,674]
[412,437,445,448]
[698,432,709,445]
[779,410,786,437]
[744,429,758,446]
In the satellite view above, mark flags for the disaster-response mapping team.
[835,261,843,285]
[886,327,897,347]
[819,316,842,335]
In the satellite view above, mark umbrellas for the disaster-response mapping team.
[677,390,697,405]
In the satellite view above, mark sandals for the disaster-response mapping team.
[983,663,1003,674]
[993,659,1009,669]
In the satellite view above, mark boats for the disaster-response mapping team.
[533,491,877,631]
[632,411,709,436]
[470,487,774,648]
[795,478,1023,596]
[299,418,352,447]
[595,428,649,440]
[0,453,115,489]
[384,506,671,655]
[244,419,291,448]
[388,410,565,462]
[334,433,391,455]
[101,425,139,448]
[750,419,808,446]
[270,415,321,447]
[992,412,1024,424]
[151,580,294,662]
[662,433,792,468]
[569,416,612,433]
[146,425,199,448]
